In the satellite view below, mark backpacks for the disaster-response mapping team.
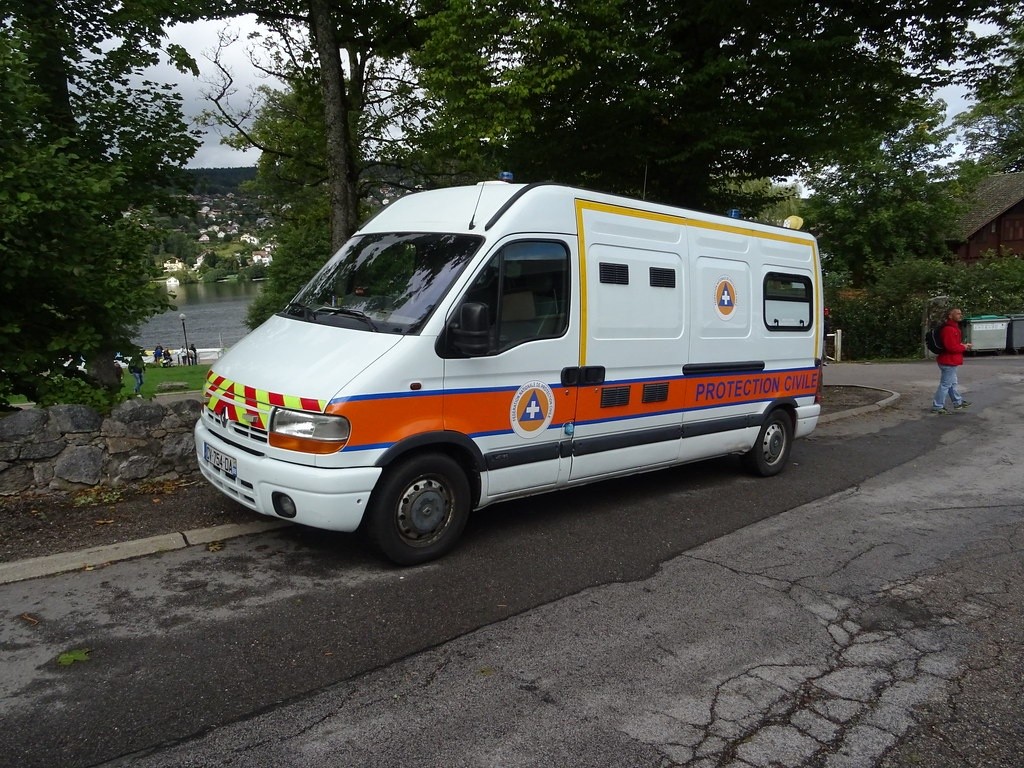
[925,324,958,355]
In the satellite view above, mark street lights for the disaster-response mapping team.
[180,313,192,366]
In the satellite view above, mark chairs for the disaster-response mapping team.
[489,290,559,348]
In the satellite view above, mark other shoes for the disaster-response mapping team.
[134,387,137,393]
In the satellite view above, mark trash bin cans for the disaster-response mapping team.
[959,315,1011,356]
[1003,313,1024,355]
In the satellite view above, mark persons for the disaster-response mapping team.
[128,353,146,398]
[153,343,197,368]
[930,306,974,415]
[113,362,125,383]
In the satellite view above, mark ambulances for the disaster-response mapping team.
[195,171,840,570]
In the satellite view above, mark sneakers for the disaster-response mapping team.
[931,407,954,416]
[954,401,975,411]
